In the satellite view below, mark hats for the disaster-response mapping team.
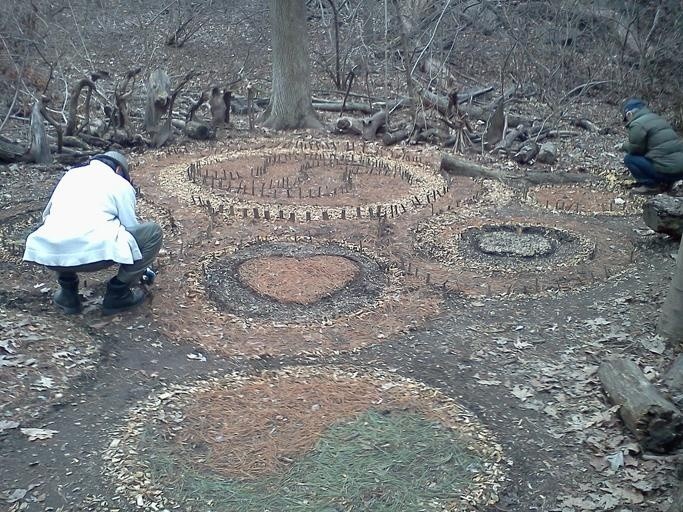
[622,99,647,121]
[92,151,130,181]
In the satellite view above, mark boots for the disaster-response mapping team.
[102,276,143,315]
[54,276,80,313]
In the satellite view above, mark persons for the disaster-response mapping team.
[614,99,683,194]
[22,150,162,316]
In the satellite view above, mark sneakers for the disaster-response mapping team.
[631,184,661,195]
